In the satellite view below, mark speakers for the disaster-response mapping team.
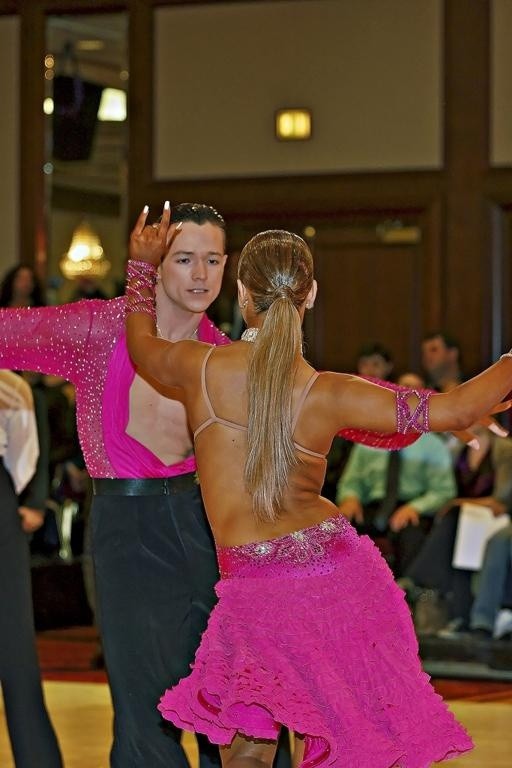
[53,75,105,163]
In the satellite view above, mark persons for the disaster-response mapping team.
[0,367,41,496]
[0,195,293,768]
[1,261,50,306]
[1,451,66,768]
[331,334,511,642]
[123,195,512,768]
[18,373,107,672]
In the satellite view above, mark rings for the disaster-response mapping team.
[151,224,160,231]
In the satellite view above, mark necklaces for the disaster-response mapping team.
[241,328,307,351]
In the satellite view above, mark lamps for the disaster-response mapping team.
[274,107,313,142]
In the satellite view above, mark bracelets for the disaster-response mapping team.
[122,261,159,314]
